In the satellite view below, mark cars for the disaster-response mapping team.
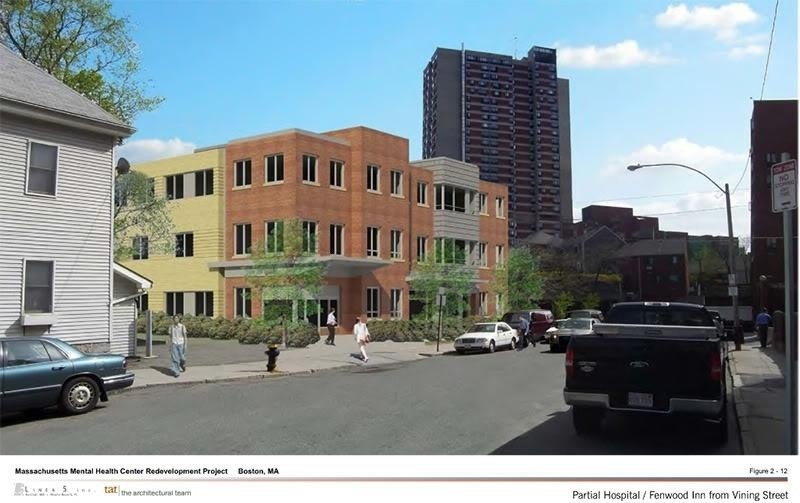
[1,332,135,414]
[549,316,602,351]
[543,317,572,341]
[454,321,522,352]
[708,309,721,325]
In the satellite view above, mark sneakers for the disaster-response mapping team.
[364,357,368,362]
[359,354,363,358]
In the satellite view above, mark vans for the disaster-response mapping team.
[566,308,604,323]
[498,306,555,345]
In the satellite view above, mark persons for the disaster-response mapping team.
[350,313,371,363]
[755,305,773,349]
[165,310,190,379]
[324,306,340,346]
[516,314,536,351]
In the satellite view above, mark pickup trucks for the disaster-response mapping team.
[564,297,739,448]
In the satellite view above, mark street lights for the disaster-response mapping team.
[626,161,743,348]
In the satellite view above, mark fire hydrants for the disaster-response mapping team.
[263,343,280,370]
